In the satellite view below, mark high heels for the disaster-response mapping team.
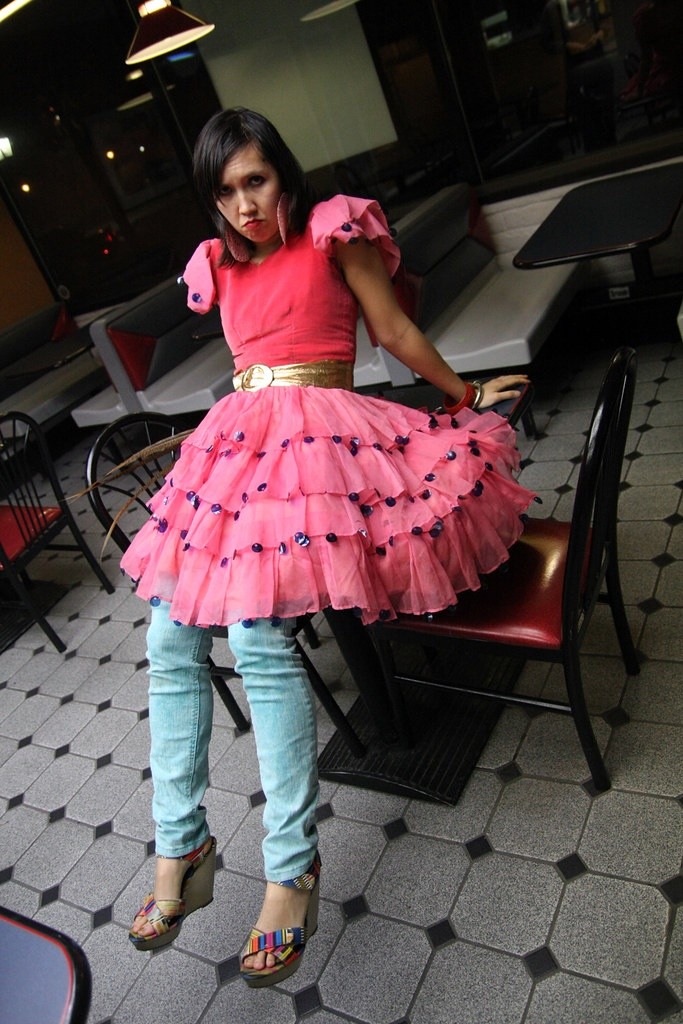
[239,850,323,989]
[128,836,217,952]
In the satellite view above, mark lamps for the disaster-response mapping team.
[123,1,216,67]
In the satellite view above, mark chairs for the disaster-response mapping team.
[84,411,370,767]
[0,407,116,658]
[358,347,641,795]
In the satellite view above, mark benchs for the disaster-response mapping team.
[2,181,582,444]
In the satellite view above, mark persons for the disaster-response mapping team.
[118,106,538,988]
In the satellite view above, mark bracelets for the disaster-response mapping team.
[444,380,483,413]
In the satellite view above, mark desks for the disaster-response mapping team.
[512,160,682,348]
[189,311,241,345]
[287,375,535,810]
[1,315,97,384]
